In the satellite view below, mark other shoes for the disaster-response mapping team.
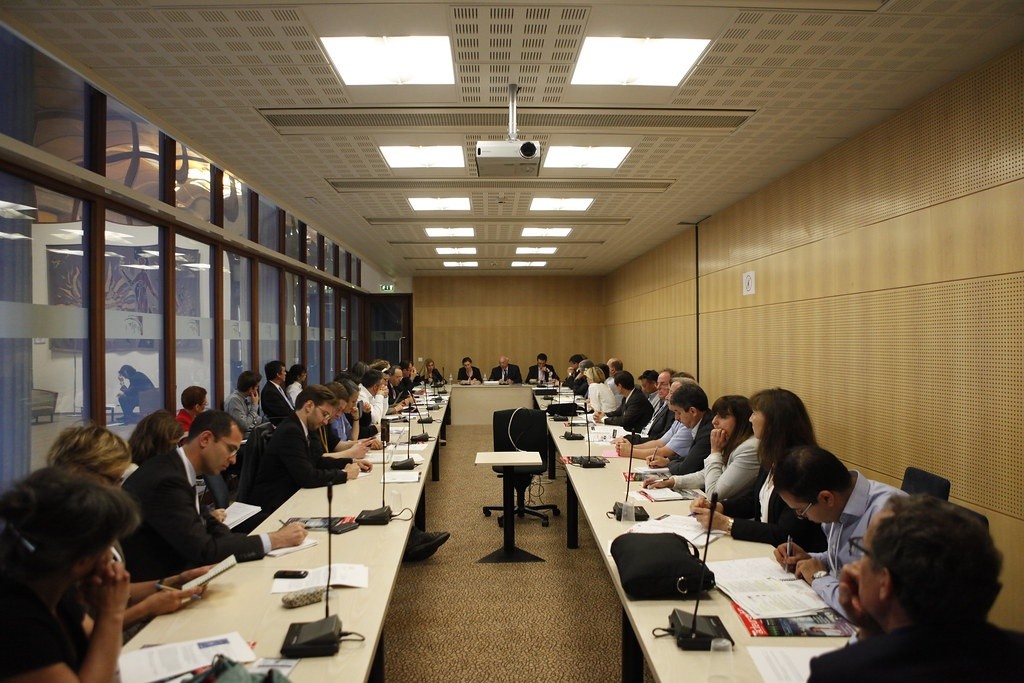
[405,527,450,562]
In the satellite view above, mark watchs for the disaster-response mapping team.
[811,570,829,579]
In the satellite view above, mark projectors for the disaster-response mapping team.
[476,140,541,179]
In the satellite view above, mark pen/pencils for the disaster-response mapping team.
[153,583,202,599]
[645,478,670,485]
[648,446,658,467]
[784,534,792,574]
[279,519,287,526]
[687,512,699,516]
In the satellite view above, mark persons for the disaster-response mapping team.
[488,355,522,385]
[808,493,1024,683]
[524,353,560,385]
[249,384,360,510]
[690,389,828,553]
[310,359,420,459]
[0,468,140,683]
[420,359,447,383]
[46,425,216,640]
[773,445,911,625]
[457,357,482,383]
[116,365,156,419]
[562,353,761,502]
[121,410,308,584]
[127,361,307,447]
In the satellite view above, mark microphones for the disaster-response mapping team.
[412,367,447,444]
[279,481,342,657]
[391,402,414,470]
[670,491,735,650]
[545,378,606,468]
[612,428,650,523]
[355,427,392,525]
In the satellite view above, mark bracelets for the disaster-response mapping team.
[353,419,359,421]
[157,578,163,591]
[727,517,735,536]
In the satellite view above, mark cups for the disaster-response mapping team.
[706,638,737,683]
[390,489,402,515]
[619,500,635,530]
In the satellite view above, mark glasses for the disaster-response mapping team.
[794,489,833,520]
[213,432,238,457]
[314,403,331,422]
[393,375,404,381]
[77,462,124,487]
[654,382,671,388]
[200,402,209,406]
[848,536,898,584]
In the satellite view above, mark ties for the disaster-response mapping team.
[504,369,508,382]
[192,484,198,498]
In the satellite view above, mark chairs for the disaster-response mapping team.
[137,387,161,418]
[199,469,230,511]
[483,407,560,528]
[234,419,276,502]
[31,389,59,423]
[900,466,949,502]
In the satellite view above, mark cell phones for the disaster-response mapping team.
[274,571,308,579]
[331,521,359,534]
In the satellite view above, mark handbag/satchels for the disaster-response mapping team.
[548,403,577,417]
[611,532,716,600]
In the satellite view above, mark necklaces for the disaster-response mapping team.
[319,427,329,453]
[828,522,844,579]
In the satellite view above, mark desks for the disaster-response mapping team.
[80,405,114,422]
[118,381,862,683]
